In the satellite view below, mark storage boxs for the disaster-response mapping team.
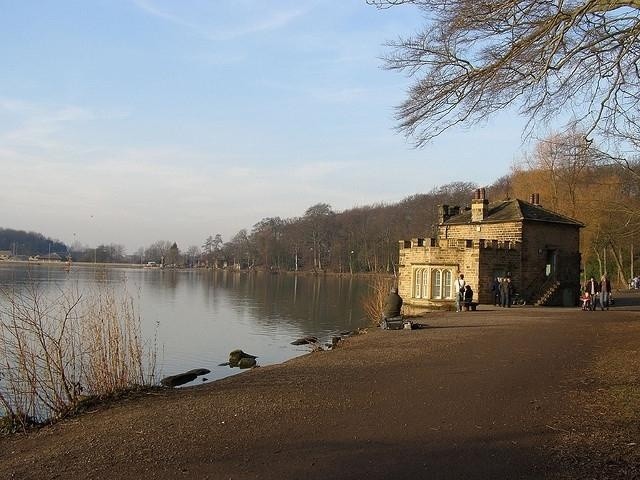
[386,315,402,329]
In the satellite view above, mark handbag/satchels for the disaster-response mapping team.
[608,298,616,306]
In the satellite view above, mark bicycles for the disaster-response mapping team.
[511,291,527,307]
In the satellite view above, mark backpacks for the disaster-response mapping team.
[459,286,465,292]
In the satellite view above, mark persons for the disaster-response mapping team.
[507,278,513,308]
[465,285,473,301]
[583,290,593,311]
[585,277,599,311]
[491,277,501,307]
[381,287,403,329]
[454,274,466,312]
[499,278,509,308]
[598,274,616,311]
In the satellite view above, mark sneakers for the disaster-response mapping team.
[455,310,470,312]
